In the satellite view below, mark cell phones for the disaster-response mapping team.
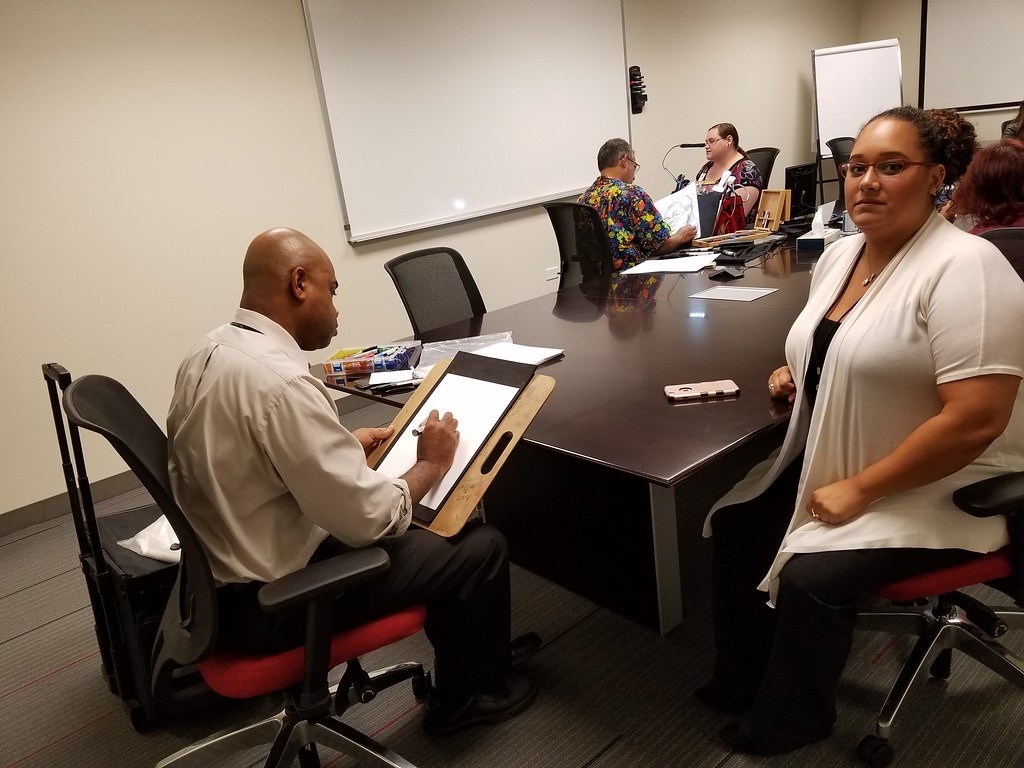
[664,380,740,401]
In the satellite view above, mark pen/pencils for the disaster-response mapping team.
[371,378,424,394]
[679,246,736,256]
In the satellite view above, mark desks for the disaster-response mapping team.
[308,219,852,638]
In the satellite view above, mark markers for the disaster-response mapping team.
[347,346,406,357]
[413,425,425,436]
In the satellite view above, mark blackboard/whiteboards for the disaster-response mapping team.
[300,0,633,242]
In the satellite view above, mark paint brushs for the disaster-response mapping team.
[631,76,646,94]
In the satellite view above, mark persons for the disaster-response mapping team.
[573,138,697,273]
[168,229,540,736]
[1002,101,1024,142]
[691,105,1024,758]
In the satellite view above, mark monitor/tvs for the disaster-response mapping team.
[785,162,818,223]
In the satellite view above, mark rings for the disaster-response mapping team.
[811,508,821,518]
[768,384,774,390]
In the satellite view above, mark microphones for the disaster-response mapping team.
[661,142,705,183]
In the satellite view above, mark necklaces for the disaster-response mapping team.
[860,246,895,286]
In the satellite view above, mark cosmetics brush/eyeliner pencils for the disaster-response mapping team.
[630,76,645,97]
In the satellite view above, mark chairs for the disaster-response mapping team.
[851,473,1024,768]
[64,373,432,768]
[542,135,860,292]
[383,247,487,336]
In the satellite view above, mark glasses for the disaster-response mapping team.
[705,138,723,146]
[839,159,937,177]
[620,156,640,174]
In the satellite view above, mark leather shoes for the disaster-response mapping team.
[422,666,538,741]
[510,632,543,667]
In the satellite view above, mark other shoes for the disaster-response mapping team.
[718,718,831,756]
[694,674,753,715]
